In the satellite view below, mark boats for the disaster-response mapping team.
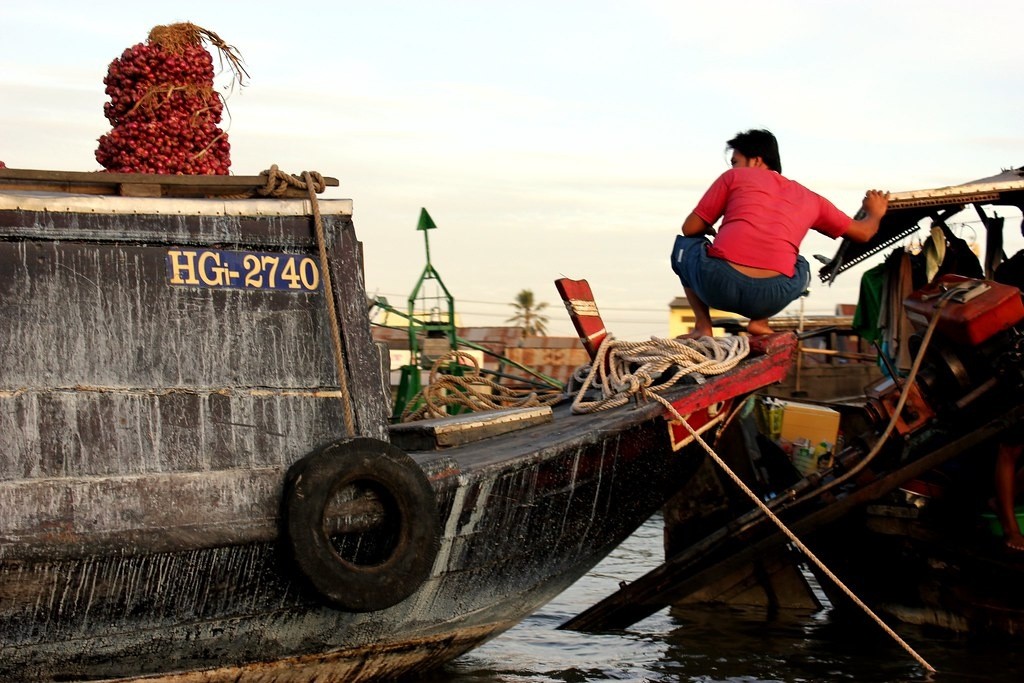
[0,162,1024,683]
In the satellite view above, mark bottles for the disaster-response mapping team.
[781,437,841,477]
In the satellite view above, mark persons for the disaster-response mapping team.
[670,129,890,341]
